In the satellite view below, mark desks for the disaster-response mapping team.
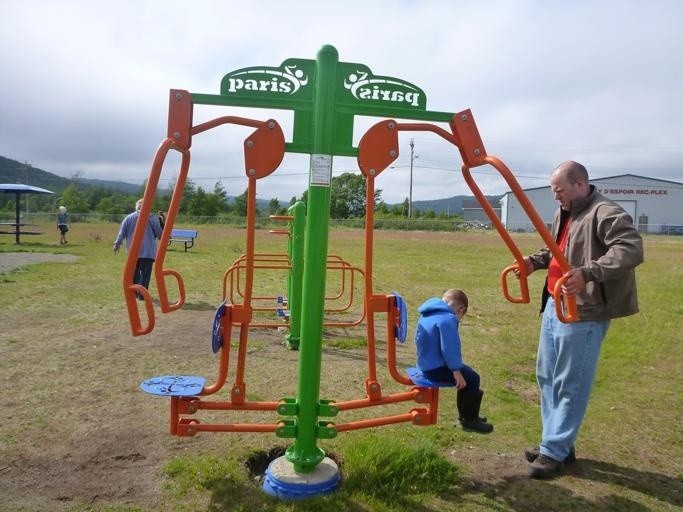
[0,223,39,243]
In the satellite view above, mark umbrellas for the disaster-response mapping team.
[0,182,54,244]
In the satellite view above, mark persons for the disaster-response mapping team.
[113,198,162,301]
[56,205,69,246]
[415,288,494,435]
[157,210,166,229]
[512,160,645,479]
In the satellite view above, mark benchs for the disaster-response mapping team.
[165,229,198,252]
[0,230,45,235]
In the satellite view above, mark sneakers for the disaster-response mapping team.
[459,416,493,435]
[525,445,575,480]
